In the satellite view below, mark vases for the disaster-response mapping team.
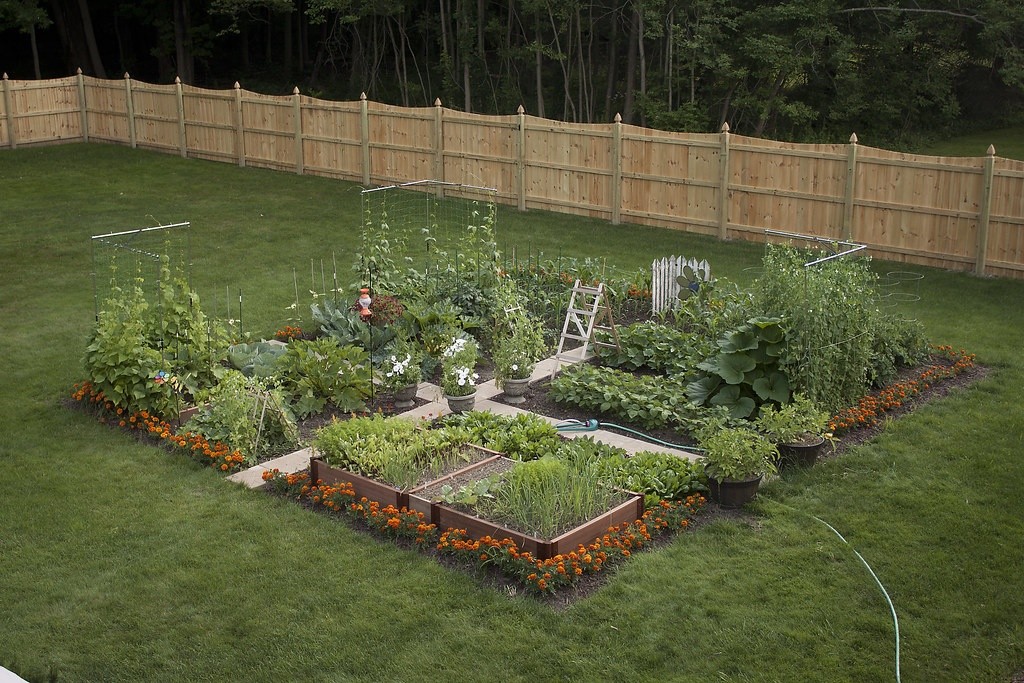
[444,388,479,413]
[502,373,532,403]
[393,382,418,407]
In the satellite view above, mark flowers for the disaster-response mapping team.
[441,365,479,396]
[493,338,535,390]
[379,352,422,392]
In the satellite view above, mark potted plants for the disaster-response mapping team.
[702,426,779,510]
[755,394,830,469]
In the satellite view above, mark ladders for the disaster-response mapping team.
[550,278,623,384]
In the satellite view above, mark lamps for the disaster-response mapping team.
[359,287,373,316]
